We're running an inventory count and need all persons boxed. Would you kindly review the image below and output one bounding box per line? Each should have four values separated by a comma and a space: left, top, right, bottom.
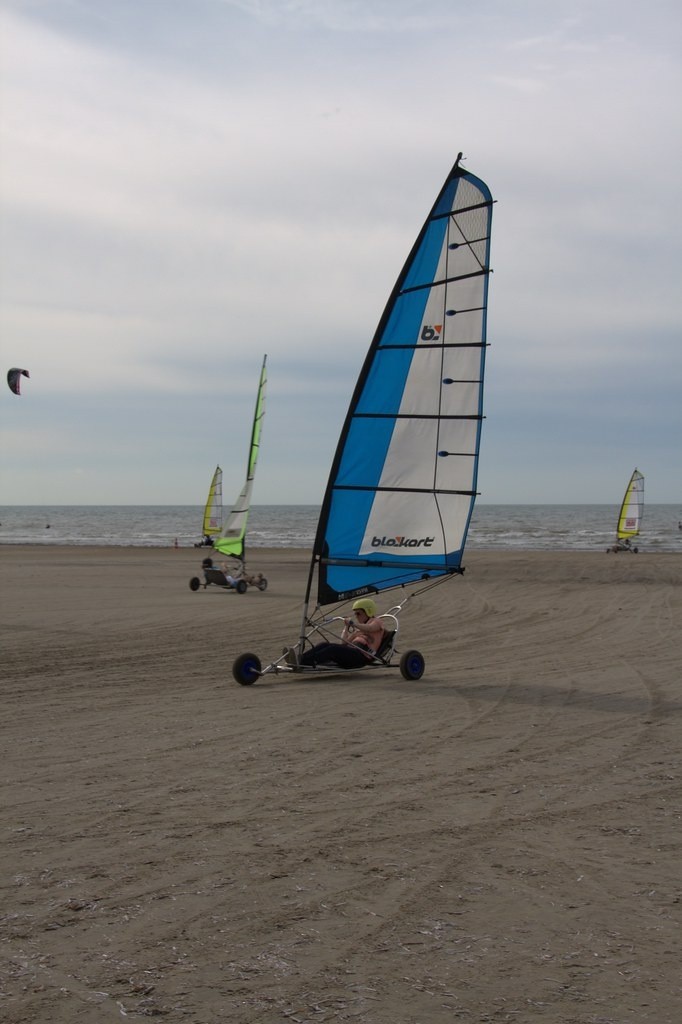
201, 556, 264, 588
197, 535, 211, 547
611, 539, 631, 552
283, 598, 387, 672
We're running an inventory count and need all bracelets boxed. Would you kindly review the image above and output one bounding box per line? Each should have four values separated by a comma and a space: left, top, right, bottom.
348, 619, 355, 627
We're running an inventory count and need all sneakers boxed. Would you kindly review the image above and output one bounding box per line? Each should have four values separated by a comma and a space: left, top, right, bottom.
282, 646, 299, 669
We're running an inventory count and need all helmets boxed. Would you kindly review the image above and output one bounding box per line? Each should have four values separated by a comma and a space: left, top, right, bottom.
353, 599, 376, 618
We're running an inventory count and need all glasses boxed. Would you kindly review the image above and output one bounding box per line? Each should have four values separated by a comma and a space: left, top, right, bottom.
354, 611, 365, 616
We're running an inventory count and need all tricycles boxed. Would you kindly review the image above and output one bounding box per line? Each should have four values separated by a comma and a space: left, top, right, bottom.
605, 466, 644, 552
193, 464, 222, 549
189, 354, 269, 591
218, 152, 500, 684
